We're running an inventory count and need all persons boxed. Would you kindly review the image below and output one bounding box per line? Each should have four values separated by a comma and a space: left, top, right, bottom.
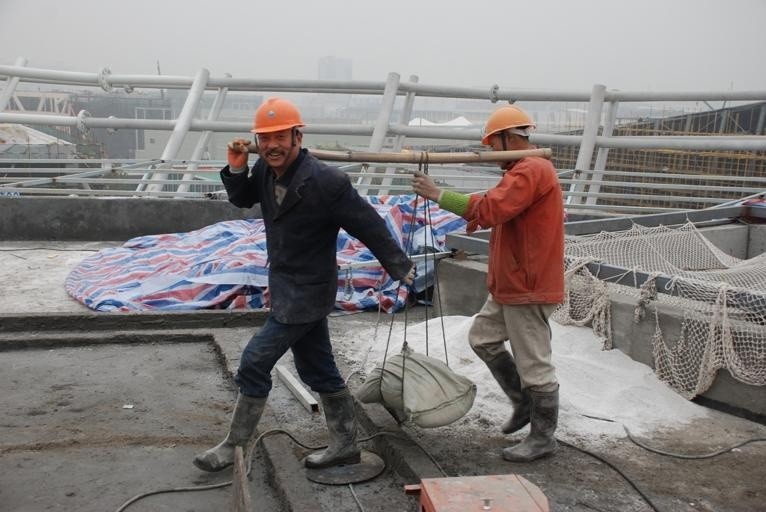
193, 97, 416, 471
412, 106, 565, 462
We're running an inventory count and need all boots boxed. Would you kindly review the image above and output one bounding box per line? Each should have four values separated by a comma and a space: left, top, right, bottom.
305, 385, 360, 469
193, 388, 268, 472
485, 351, 530, 433
502, 384, 560, 462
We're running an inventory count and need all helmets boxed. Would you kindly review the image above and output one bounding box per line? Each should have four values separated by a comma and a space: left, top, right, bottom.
482, 106, 535, 144
250, 97, 305, 133
